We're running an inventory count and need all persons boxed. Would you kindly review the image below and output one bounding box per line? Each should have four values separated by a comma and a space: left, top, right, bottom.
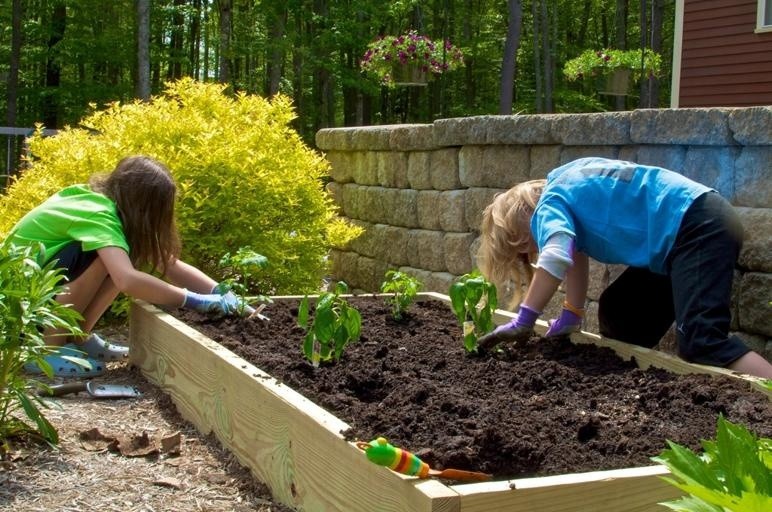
466, 157, 771, 385
1, 154, 272, 379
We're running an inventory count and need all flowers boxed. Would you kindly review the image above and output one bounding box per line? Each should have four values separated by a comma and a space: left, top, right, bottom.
359, 29, 467, 90
562, 47, 664, 82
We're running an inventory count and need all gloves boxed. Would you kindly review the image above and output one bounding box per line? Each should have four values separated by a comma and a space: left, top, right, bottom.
479, 304, 543, 340
545, 301, 587, 336
181, 284, 268, 320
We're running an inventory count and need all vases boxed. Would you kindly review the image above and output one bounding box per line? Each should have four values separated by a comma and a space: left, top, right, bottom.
605, 70, 629, 94
392, 64, 426, 83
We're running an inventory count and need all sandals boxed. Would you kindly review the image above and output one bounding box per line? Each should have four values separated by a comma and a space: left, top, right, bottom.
26, 333, 129, 377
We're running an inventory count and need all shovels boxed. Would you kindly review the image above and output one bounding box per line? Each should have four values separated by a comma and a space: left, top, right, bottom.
358, 438, 494, 482
37, 381, 141, 399
